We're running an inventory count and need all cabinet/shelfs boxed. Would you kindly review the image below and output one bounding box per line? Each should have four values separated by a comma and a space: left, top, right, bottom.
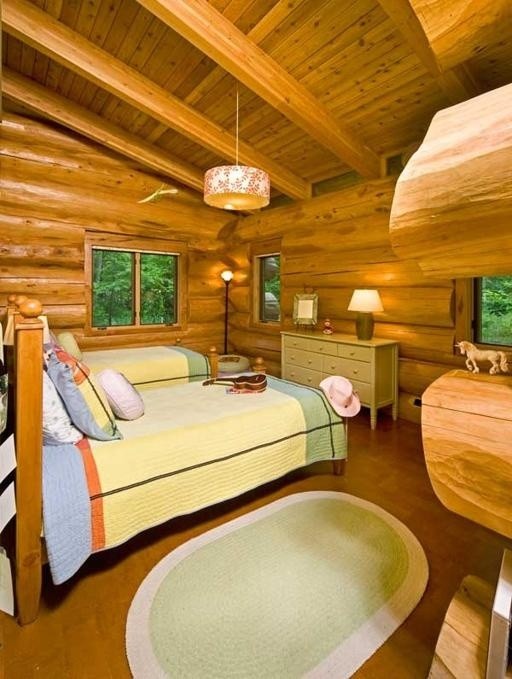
278, 331, 400, 431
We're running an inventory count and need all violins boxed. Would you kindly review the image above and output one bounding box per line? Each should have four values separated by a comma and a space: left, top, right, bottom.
203, 375, 267, 389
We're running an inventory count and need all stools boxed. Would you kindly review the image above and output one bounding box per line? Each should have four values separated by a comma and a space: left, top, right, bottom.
215, 354, 251, 378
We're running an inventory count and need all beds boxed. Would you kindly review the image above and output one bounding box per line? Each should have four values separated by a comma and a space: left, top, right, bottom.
12, 295, 346, 627
6, 294, 219, 396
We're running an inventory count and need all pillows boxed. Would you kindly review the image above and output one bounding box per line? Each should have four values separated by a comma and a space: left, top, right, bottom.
57, 332, 83, 362
50, 329, 59, 344
41, 343, 125, 441
97, 368, 145, 421
42, 370, 84, 446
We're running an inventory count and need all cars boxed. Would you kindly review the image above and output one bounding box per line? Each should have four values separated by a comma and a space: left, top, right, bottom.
265, 292, 279, 321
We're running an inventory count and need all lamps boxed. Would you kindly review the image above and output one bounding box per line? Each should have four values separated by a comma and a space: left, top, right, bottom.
346, 289, 384, 342
220, 270, 234, 353
203, 85, 271, 211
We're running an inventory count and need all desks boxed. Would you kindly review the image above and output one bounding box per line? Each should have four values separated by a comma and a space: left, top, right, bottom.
420, 371, 511, 538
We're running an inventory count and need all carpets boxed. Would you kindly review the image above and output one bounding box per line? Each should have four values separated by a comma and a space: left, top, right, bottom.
125, 489, 430, 679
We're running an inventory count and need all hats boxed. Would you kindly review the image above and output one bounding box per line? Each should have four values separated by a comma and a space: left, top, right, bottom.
318, 375, 361, 418
